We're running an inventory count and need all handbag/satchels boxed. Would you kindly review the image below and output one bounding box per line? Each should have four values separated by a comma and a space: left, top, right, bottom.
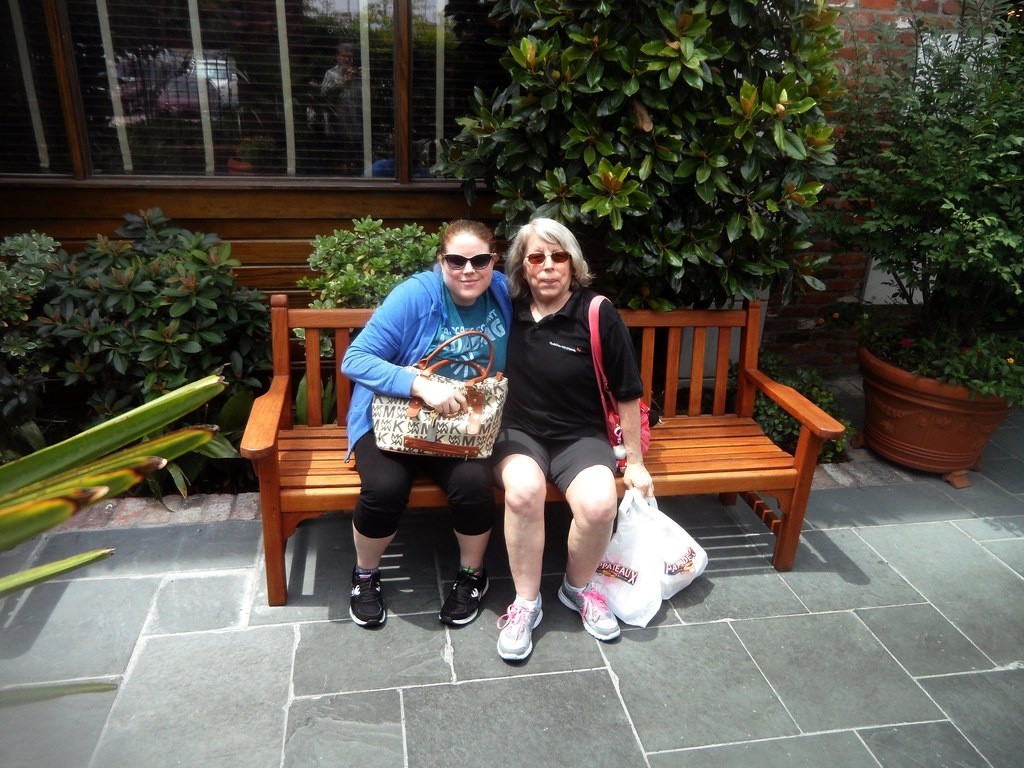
591, 488, 710, 627
372, 331, 508, 459
588, 295, 650, 473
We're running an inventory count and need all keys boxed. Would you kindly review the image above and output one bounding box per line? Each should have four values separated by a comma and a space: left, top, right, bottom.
429, 411, 439, 427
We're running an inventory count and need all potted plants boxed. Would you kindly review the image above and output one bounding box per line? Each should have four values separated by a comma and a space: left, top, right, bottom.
830, 0, 1024, 474
226, 137, 276, 174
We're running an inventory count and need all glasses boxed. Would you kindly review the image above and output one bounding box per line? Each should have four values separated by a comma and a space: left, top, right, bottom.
441, 253, 493, 270
524, 252, 571, 264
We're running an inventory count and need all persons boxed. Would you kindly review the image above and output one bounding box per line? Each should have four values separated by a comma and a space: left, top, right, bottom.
489, 216, 655, 660
319, 41, 366, 143
340, 218, 515, 627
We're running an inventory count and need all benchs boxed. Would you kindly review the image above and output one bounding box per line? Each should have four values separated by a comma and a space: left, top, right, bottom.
241, 297, 847, 608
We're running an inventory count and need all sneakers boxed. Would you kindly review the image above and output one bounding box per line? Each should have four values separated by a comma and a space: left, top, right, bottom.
558, 573, 621, 644
438, 566, 490, 630
349, 561, 386, 629
497, 591, 544, 665
